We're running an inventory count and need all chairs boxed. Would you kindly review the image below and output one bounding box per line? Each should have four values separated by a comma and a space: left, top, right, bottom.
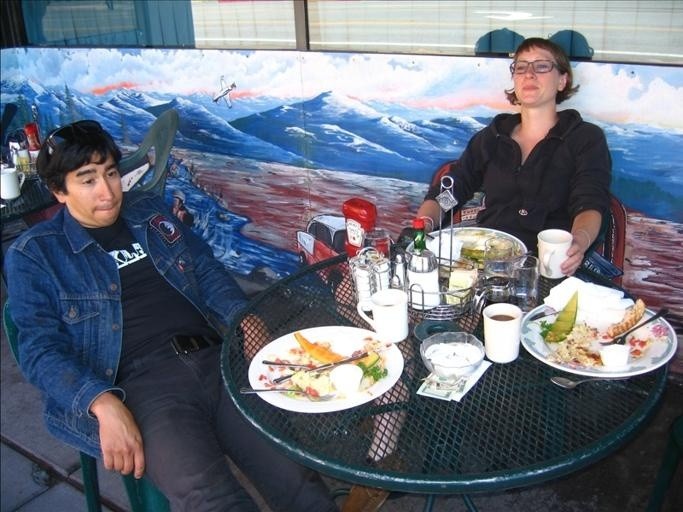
111, 107, 180, 201
429, 157, 627, 292
1, 291, 173, 511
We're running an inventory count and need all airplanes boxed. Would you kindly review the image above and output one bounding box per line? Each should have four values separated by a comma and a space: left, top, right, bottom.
210, 73, 236, 109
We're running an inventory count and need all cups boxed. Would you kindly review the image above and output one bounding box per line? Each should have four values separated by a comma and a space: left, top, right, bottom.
481, 237, 540, 308
330, 363, 364, 398
482, 303, 522, 365
538, 228, 572, 280
598, 344, 630, 367
356, 288, 408, 344
0, 167, 25, 201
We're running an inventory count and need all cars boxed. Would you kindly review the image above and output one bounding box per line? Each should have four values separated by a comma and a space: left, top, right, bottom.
294, 209, 392, 291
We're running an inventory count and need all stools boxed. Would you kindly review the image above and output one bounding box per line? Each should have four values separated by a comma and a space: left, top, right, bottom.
643, 414, 682, 512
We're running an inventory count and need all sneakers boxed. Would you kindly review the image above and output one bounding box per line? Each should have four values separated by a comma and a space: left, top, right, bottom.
357, 413, 376, 435
337, 448, 413, 512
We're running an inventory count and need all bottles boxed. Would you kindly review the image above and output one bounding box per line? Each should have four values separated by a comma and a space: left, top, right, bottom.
349, 218, 440, 311
7, 121, 44, 178
340, 197, 378, 260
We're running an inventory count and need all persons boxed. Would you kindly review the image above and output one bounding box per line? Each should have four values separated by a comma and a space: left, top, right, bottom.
3, 118, 337, 512
416, 37, 614, 277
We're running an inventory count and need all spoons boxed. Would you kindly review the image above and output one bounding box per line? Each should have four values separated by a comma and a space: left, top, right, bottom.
548, 374, 601, 389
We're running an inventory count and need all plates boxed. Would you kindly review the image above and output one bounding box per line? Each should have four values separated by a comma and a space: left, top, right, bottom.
246, 324, 406, 412
518, 303, 678, 379
403, 227, 529, 272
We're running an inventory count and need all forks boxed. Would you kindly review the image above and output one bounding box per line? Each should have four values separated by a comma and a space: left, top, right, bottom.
238, 387, 334, 402
600, 308, 668, 346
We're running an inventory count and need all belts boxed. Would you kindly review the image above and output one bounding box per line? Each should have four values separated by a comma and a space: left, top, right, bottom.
112, 326, 227, 385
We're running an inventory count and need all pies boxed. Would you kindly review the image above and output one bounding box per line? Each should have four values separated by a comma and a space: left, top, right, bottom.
606, 298, 646, 338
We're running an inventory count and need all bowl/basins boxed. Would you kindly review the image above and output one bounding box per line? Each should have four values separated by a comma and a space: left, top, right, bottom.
418, 331, 484, 378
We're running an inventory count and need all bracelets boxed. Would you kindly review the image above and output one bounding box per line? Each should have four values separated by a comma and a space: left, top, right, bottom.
419, 215, 434, 229
573, 229, 591, 248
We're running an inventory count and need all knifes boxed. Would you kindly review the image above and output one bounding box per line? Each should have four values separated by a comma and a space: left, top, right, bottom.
529, 306, 560, 321
272, 349, 372, 383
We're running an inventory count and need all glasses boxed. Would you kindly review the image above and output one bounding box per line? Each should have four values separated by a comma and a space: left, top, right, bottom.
41, 119, 107, 157
508, 57, 565, 77
